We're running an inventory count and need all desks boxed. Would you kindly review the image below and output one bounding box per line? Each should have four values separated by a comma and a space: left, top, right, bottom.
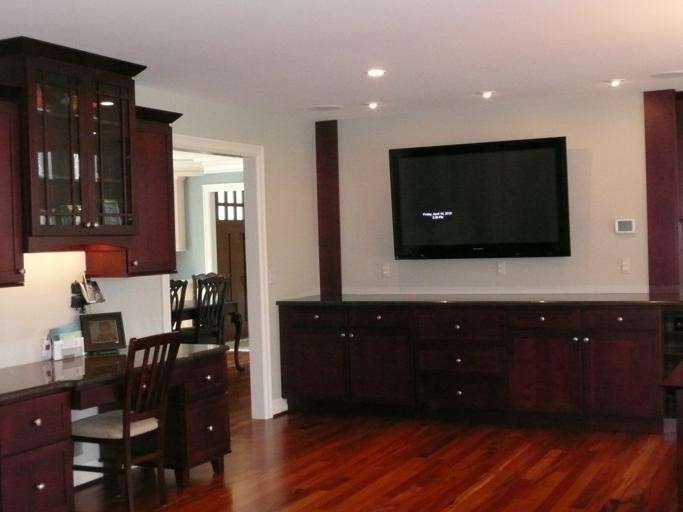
0, 340, 232, 510
169, 299, 245, 372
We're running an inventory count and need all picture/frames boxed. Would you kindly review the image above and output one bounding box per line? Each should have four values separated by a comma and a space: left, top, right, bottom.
77, 311, 126, 353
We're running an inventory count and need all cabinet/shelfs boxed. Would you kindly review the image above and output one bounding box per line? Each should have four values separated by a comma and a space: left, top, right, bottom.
275, 292, 683, 435
0, 36, 182, 290
0, 390, 74, 511
97, 354, 232, 487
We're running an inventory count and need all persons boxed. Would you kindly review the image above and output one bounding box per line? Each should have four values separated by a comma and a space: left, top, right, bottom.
94, 323, 113, 341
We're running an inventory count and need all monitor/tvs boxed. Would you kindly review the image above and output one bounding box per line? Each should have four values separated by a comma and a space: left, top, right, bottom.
390, 135, 572, 261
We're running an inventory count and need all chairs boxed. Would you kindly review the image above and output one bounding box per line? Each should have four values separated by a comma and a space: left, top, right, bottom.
169, 272, 231, 345
70, 329, 185, 511
